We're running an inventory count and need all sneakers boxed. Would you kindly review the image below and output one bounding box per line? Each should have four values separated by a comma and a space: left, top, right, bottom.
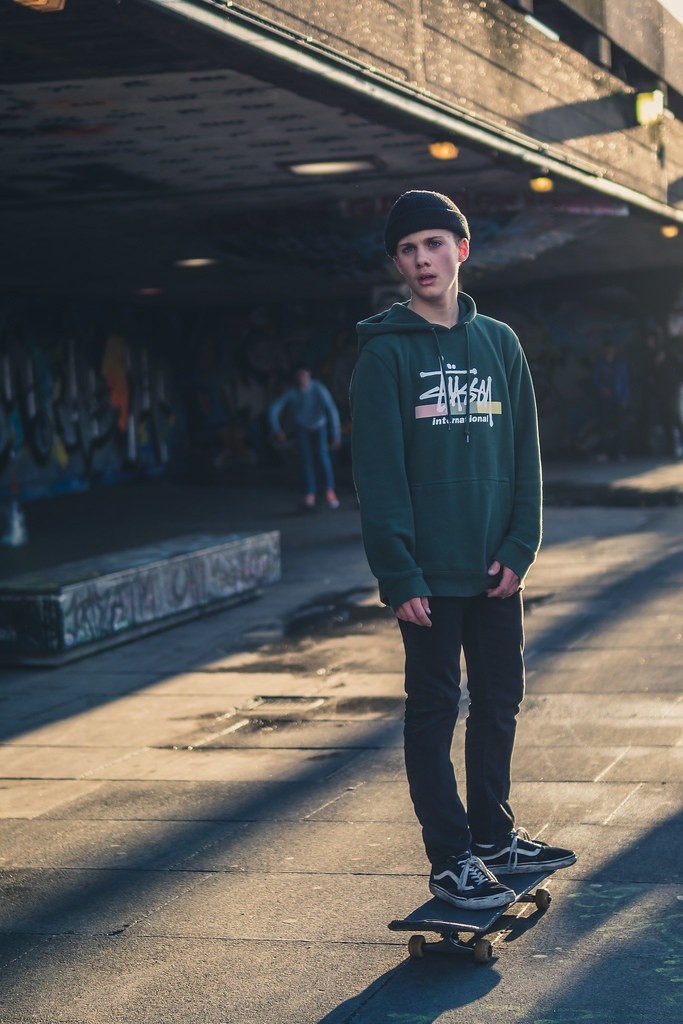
472, 828, 576, 875
428, 848, 515, 909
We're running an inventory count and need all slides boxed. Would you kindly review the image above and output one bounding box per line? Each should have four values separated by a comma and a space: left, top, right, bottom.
387, 840, 558, 963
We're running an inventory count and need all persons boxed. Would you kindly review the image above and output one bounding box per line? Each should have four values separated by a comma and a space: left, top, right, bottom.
268, 364, 342, 511
349, 190, 578, 910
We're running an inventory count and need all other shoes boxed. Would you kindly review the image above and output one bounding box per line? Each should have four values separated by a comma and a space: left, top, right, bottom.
327, 494, 338, 509
306, 494, 315, 507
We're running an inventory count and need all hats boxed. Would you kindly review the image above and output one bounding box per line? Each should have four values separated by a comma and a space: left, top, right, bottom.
385, 189, 470, 258
